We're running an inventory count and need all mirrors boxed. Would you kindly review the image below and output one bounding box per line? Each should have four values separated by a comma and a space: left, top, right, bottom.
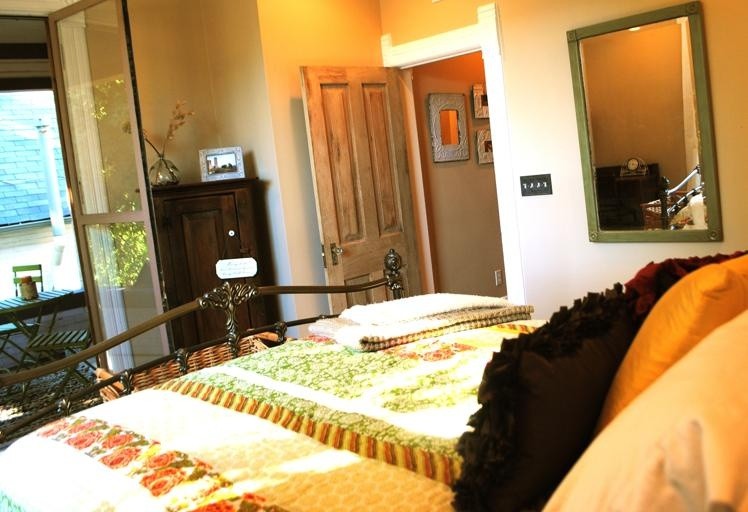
427, 90, 471, 164
565, 0, 721, 249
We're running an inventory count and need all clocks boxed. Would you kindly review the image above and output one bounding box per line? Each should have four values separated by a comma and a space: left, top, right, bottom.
619, 157, 649, 180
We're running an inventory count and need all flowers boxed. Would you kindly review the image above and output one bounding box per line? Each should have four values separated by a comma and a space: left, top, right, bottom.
120, 95, 199, 184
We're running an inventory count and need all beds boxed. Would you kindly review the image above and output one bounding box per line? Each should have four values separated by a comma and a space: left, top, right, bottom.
0, 249, 550, 511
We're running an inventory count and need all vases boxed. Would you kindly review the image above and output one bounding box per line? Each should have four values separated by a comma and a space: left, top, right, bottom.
150, 155, 180, 188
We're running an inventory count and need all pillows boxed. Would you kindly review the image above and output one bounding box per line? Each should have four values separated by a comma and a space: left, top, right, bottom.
540, 310, 748, 511
599, 255, 745, 427
446, 250, 733, 510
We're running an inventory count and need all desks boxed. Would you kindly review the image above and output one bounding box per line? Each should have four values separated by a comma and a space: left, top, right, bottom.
595, 175, 664, 229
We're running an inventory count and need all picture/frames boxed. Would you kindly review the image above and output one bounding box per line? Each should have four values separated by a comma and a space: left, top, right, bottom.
475, 126, 494, 164
197, 146, 247, 184
471, 82, 491, 120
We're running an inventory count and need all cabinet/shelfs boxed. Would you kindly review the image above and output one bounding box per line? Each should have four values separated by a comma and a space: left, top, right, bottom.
149, 178, 285, 350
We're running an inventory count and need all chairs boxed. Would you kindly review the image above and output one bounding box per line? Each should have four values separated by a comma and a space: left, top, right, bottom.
0, 259, 110, 450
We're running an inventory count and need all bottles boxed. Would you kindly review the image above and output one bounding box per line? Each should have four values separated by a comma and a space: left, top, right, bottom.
19, 276, 38, 300
